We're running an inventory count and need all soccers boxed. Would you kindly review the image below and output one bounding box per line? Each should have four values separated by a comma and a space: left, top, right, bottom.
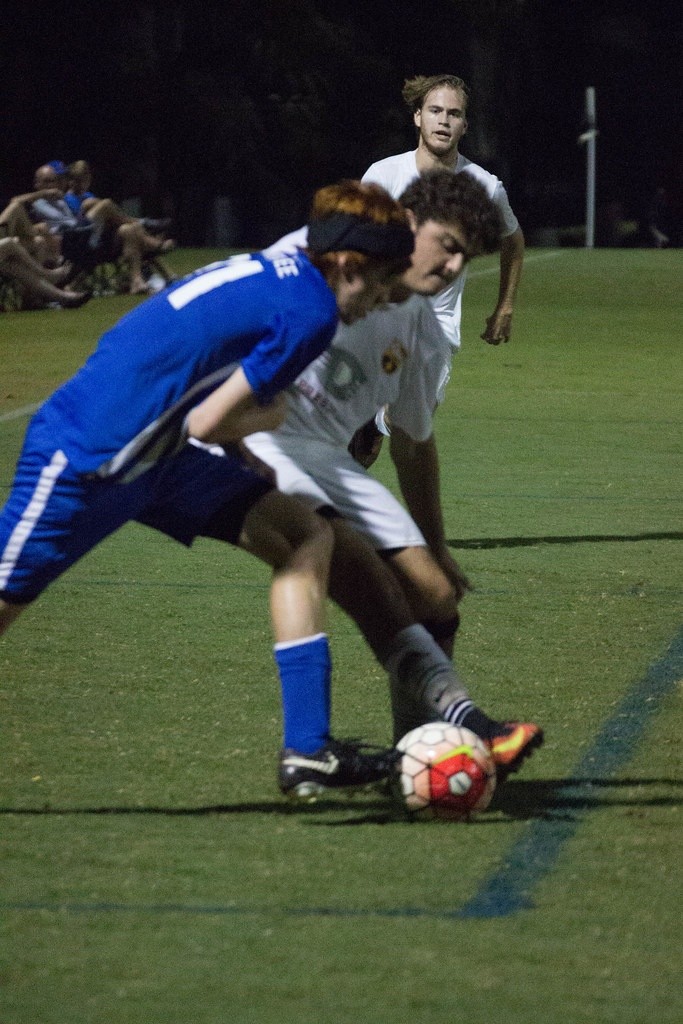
388, 720, 498, 820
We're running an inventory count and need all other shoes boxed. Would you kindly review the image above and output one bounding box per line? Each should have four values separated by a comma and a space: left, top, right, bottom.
150, 239, 176, 257
130, 277, 148, 293
57, 260, 85, 288
144, 217, 173, 235
62, 289, 92, 308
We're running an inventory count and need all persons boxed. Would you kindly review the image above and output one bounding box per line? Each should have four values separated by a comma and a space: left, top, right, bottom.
243, 167, 546, 789
1, 182, 416, 805
0, 159, 176, 314
345, 75, 526, 599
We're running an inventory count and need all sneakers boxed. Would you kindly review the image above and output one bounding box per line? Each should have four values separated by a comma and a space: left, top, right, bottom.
352, 418, 383, 469
485, 720, 544, 786
276, 738, 396, 807
